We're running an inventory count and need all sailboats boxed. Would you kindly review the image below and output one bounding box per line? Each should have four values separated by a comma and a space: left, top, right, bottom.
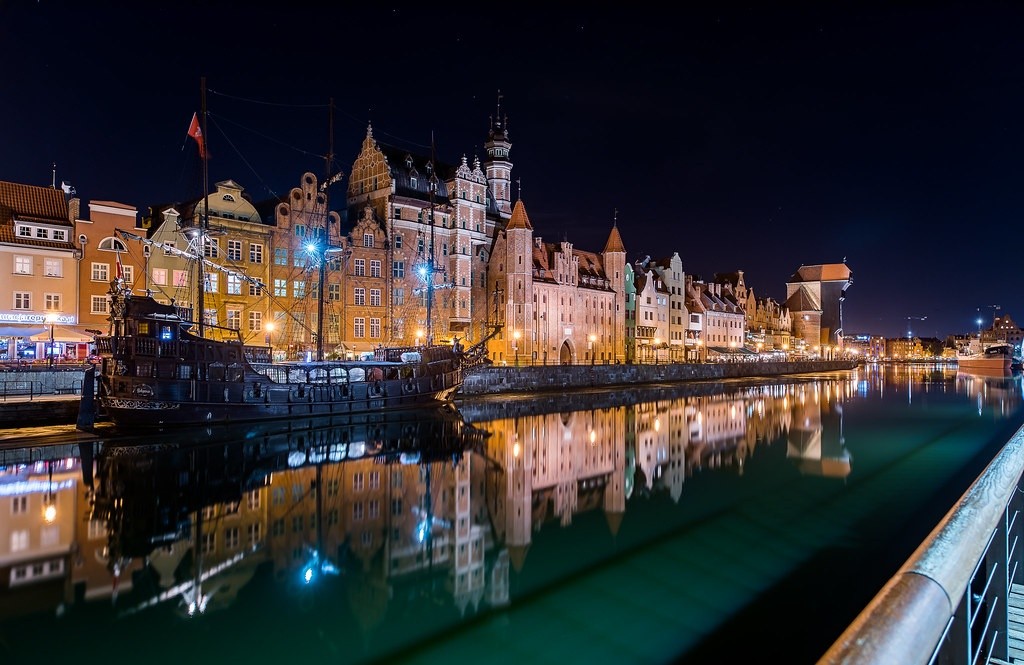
75, 77, 505, 446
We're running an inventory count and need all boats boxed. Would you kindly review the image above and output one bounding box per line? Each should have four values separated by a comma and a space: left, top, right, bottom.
92, 399, 503, 609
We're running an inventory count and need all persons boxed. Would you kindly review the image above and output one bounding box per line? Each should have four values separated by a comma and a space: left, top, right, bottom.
60, 346, 73, 361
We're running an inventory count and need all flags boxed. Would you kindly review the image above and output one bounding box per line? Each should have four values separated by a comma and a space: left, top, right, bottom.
115, 255, 122, 280
188, 116, 210, 159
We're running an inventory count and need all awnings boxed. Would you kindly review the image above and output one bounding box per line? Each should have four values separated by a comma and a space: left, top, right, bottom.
708, 347, 759, 356
0, 327, 45, 336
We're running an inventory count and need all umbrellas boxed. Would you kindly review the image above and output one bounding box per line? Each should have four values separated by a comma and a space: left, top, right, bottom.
30, 328, 95, 363
